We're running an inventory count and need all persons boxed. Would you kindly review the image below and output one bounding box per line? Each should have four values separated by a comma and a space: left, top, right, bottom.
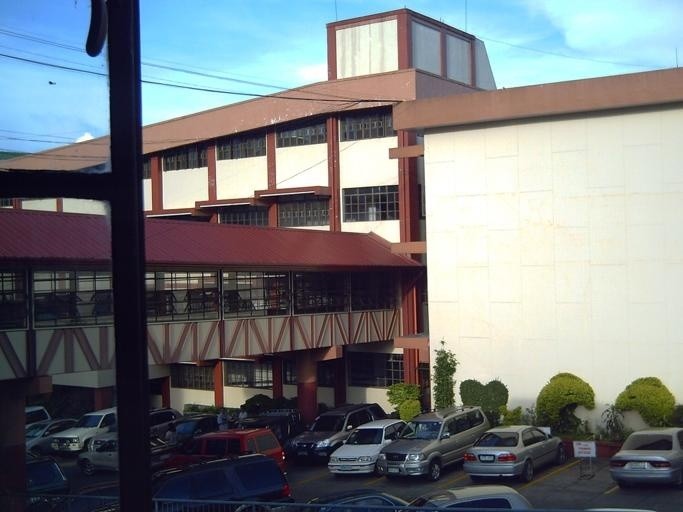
216, 407, 235, 431
238, 405, 248, 427
165, 423, 178, 441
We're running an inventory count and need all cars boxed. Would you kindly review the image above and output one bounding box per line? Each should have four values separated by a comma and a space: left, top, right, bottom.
16, 395, 305, 476
298, 491, 410, 512
609, 428, 682, 491
328, 420, 415, 474
462, 425, 567, 483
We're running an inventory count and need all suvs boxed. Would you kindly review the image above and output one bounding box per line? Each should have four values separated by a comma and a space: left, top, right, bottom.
145, 454, 294, 512
376, 405, 493, 479
397, 484, 533, 510
290, 404, 389, 460
163, 426, 287, 471
